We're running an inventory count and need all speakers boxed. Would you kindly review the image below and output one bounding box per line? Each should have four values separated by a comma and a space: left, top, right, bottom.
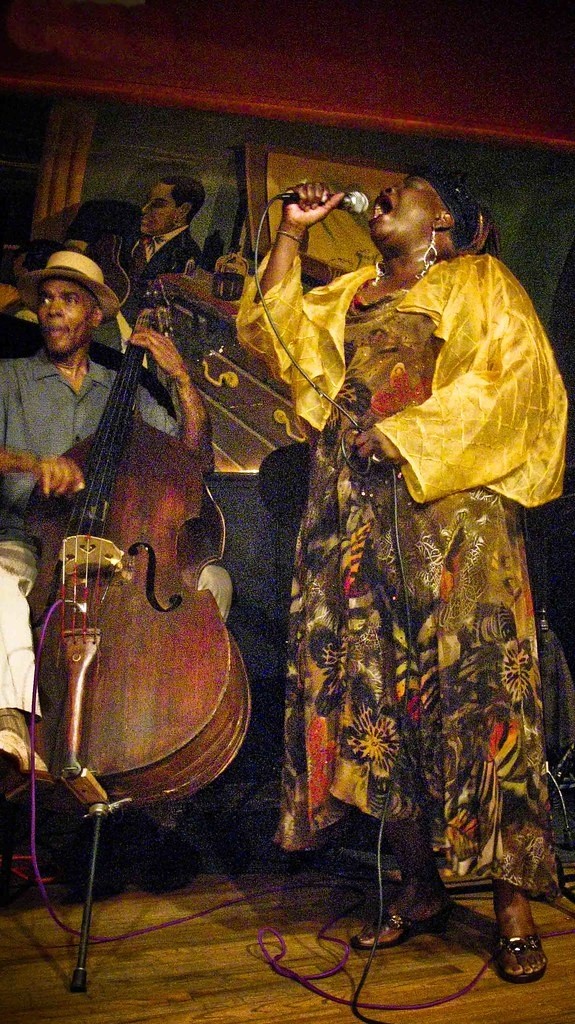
185, 470, 301, 812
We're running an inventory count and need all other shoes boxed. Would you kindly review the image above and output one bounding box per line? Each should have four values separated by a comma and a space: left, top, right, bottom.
0, 729, 53, 807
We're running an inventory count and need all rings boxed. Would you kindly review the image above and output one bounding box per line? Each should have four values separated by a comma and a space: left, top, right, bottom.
372, 453, 385, 463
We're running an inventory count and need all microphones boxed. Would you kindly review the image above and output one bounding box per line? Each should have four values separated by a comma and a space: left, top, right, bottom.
276, 191, 370, 215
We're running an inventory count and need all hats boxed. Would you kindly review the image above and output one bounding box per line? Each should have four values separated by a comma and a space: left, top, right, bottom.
17, 250, 119, 325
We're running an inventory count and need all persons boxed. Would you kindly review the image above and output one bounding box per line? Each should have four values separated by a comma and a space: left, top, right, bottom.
0, 252, 233, 786
234, 167, 570, 983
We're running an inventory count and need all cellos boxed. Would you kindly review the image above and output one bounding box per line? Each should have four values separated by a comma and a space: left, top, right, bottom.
28, 279, 253, 993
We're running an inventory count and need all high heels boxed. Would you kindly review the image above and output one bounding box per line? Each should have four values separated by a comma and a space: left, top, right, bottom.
350, 899, 458, 950
493, 930, 548, 985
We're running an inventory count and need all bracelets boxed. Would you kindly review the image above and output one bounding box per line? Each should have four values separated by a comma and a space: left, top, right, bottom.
277, 229, 302, 243
